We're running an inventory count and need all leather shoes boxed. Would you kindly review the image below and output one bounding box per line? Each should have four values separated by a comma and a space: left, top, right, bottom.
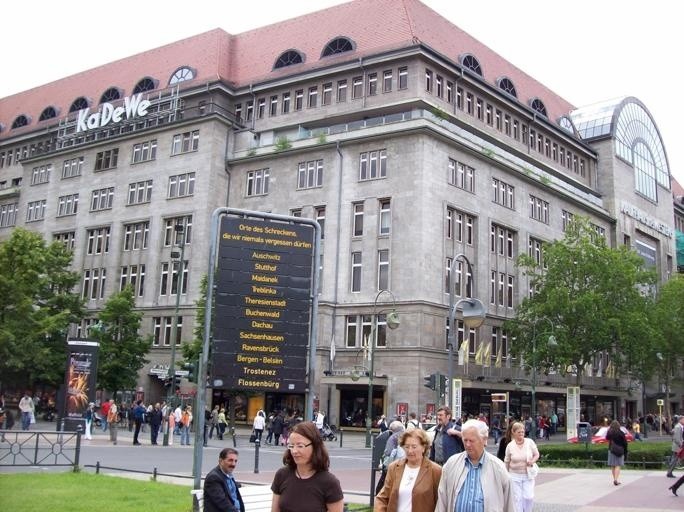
668, 475, 676, 478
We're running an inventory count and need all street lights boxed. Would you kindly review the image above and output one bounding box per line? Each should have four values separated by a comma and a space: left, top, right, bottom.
447, 250, 486, 418
368, 285, 400, 511
162, 216, 189, 405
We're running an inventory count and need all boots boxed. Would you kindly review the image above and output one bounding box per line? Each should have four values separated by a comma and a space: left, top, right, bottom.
669, 478, 684, 496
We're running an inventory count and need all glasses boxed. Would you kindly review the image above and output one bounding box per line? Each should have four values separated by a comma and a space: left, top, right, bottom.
288, 442, 313, 450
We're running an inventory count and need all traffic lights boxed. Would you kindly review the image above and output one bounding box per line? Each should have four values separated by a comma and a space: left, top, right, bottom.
164, 376, 172, 388
424, 374, 435, 390
444, 377, 448, 395
174, 374, 181, 392
398, 404, 406, 415
427, 404, 435, 413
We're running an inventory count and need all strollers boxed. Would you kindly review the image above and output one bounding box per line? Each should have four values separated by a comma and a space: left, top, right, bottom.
322, 423, 337, 442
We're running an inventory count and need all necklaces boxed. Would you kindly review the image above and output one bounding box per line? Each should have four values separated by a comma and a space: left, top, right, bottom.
296, 469, 302, 480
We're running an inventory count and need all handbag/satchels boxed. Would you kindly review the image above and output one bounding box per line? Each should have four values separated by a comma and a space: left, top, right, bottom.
610, 440, 624, 457
526, 462, 540, 480
250, 435, 256, 442
635, 432, 639, 439
383, 445, 406, 467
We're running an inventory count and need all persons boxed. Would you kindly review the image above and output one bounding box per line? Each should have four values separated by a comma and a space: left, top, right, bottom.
503, 422, 540, 512
666, 416, 684, 479
253, 406, 330, 446
632, 411, 666, 441
603, 419, 629, 486
372, 429, 442, 512
433, 418, 515, 512
32, 394, 40, 412
667, 475, 684, 497
84, 397, 230, 447
270, 420, 345, 512
18, 394, 38, 431
203, 448, 245, 512
374, 405, 559, 471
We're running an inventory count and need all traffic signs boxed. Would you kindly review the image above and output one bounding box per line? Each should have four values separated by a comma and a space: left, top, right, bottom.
206, 217, 314, 391
491, 393, 507, 402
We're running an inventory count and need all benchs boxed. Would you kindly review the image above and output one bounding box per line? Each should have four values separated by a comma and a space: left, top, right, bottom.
190, 484, 272, 512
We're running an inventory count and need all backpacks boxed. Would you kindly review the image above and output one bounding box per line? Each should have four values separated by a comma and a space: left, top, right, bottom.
410, 420, 419, 428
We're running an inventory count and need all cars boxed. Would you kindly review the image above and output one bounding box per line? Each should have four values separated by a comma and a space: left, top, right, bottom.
566, 425, 633, 446
